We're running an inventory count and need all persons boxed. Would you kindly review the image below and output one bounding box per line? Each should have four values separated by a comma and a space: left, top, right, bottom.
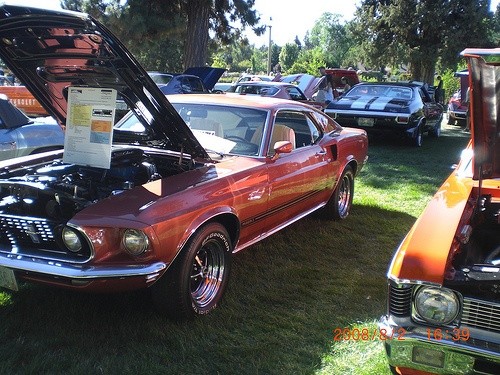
463, 87, 470, 132
436, 75, 443, 104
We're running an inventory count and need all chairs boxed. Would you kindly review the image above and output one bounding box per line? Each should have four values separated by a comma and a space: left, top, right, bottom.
189, 118, 223, 140
249, 123, 296, 156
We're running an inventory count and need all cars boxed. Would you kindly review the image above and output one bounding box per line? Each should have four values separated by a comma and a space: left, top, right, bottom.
0, 65, 473, 148
380, 45, 500, 375
1, 3, 369, 319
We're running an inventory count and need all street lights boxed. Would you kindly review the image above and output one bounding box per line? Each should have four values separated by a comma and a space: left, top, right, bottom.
263, 25, 273, 75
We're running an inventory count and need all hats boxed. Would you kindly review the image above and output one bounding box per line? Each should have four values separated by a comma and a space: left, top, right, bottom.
318, 67, 325, 69
